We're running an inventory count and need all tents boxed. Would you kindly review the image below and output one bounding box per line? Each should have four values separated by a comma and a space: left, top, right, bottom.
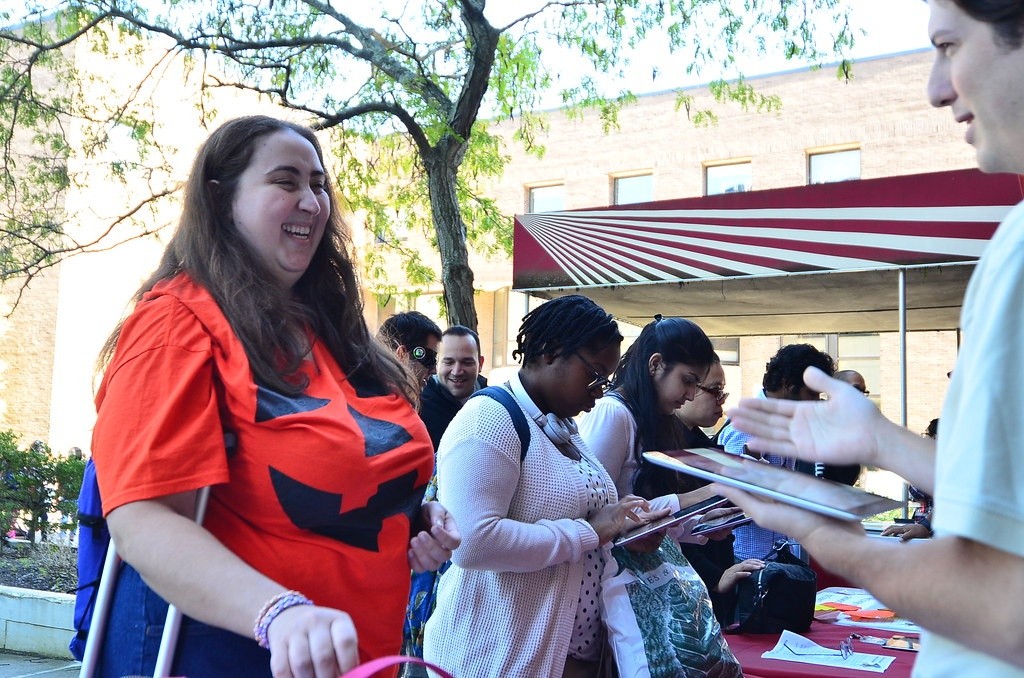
511, 167, 1024, 521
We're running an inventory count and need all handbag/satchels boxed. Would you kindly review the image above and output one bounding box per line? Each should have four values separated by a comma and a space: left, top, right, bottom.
603, 522, 744, 678
719, 539, 820, 634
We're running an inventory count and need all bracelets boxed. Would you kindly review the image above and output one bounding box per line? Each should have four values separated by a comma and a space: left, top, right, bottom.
919, 519, 935, 537
254, 590, 314, 649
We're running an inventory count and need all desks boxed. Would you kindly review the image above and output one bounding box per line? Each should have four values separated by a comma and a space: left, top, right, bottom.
726, 585, 923, 676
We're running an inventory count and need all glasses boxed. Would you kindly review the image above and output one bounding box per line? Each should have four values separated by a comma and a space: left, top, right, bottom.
698, 381, 725, 402
853, 384, 868, 393
920, 431, 928, 437
574, 350, 615, 395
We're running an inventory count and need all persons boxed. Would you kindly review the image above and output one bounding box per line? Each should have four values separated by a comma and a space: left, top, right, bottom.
91, 116, 461, 678
375, 313, 941, 630
421, 294, 723, 678
712, 0, 1024, 678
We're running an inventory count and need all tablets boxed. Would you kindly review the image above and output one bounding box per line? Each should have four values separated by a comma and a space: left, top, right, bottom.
691, 511, 754, 536
642, 446, 907, 522
615, 495, 729, 547
